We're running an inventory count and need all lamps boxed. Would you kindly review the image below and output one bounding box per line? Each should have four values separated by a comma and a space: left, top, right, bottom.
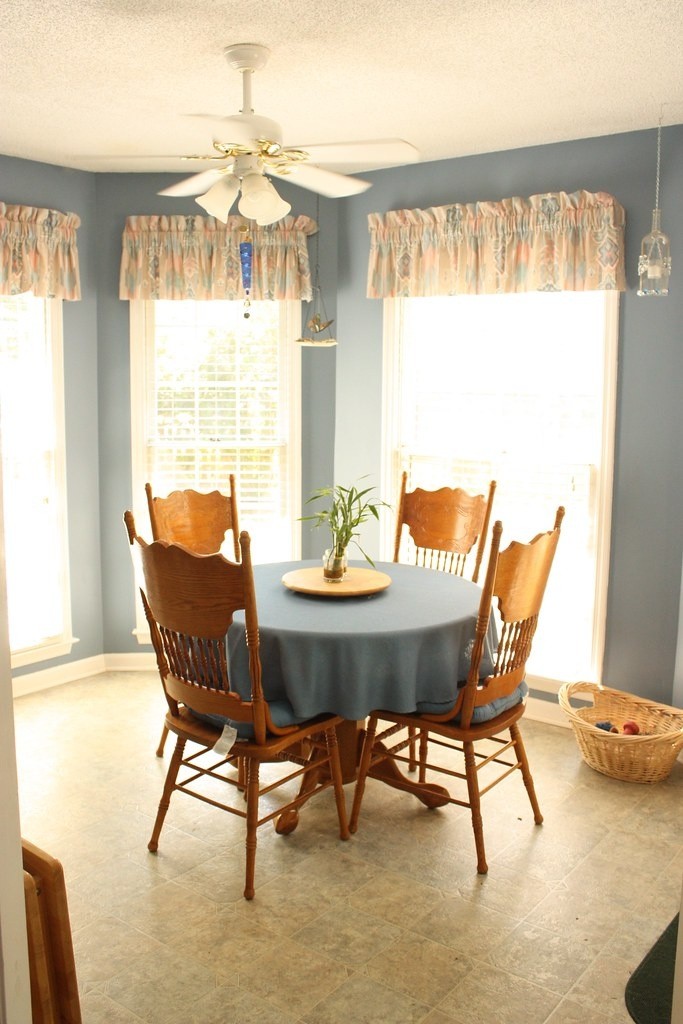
637, 116, 672, 297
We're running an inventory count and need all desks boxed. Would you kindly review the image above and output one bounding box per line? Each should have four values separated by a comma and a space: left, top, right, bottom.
173, 558, 498, 836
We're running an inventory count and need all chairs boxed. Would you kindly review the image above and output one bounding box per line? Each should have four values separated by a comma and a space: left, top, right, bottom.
123, 470, 565, 903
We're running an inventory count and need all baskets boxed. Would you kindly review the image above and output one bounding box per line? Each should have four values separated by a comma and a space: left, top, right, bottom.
558, 681, 683, 784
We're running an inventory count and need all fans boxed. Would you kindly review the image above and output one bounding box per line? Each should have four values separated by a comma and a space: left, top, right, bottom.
72, 40, 422, 227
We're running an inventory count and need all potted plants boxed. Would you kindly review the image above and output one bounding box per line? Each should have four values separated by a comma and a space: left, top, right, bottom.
296, 473, 392, 583
